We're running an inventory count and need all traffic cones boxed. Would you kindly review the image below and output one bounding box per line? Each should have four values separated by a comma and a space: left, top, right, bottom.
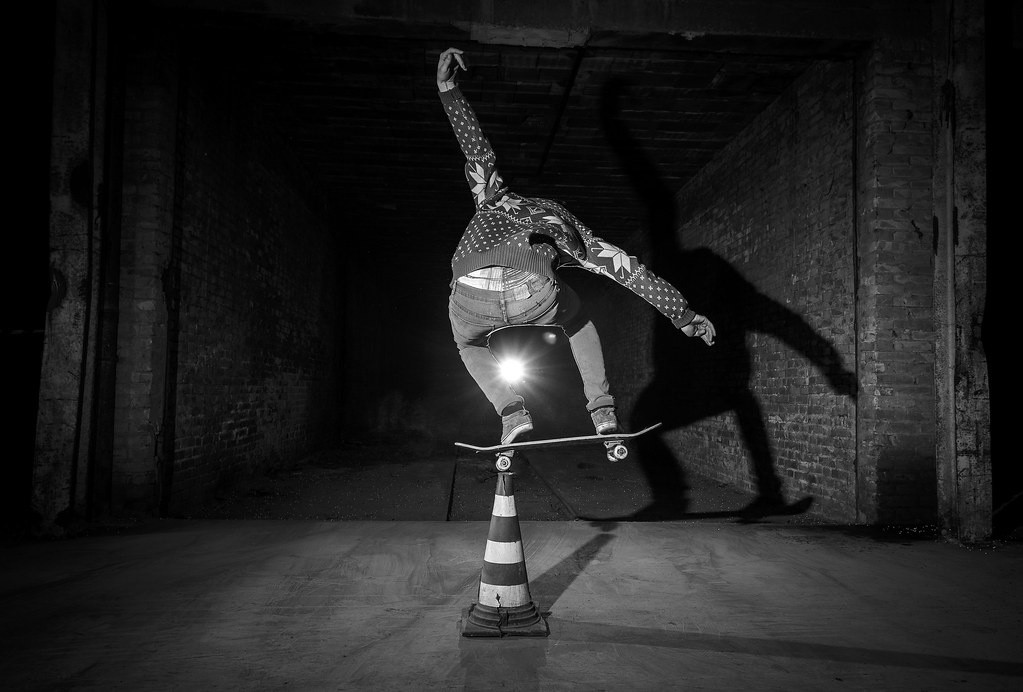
461, 470, 551, 638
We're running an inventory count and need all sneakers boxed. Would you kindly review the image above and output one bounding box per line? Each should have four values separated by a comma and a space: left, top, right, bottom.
590, 407, 617, 434
500, 410, 533, 445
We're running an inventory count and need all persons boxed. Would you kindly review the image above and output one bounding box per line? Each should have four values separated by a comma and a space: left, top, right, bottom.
435, 46, 716, 447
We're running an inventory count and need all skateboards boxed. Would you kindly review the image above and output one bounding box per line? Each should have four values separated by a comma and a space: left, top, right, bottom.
453, 420, 665, 472
